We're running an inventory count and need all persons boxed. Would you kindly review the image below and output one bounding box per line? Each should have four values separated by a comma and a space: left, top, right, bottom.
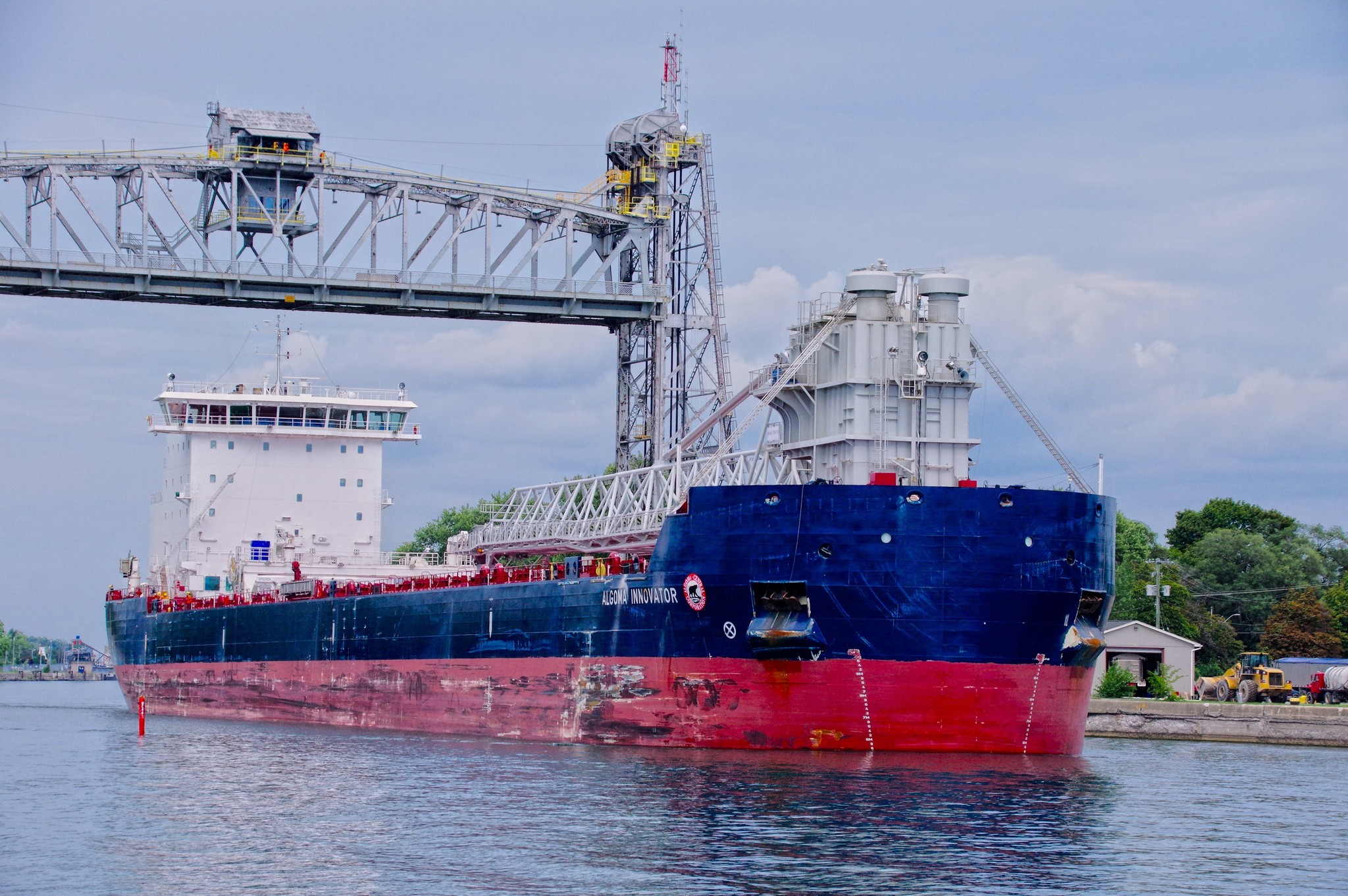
110, 585, 113, 593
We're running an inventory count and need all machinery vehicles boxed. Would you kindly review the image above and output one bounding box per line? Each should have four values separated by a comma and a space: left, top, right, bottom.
1195, 653, 1292, 703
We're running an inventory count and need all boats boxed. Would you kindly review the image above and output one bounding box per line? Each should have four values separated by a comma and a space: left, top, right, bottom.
101, 257, 1117, 754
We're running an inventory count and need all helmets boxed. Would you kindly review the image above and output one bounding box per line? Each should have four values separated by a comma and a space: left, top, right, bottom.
164, 593, 167, 596
186, 592, 190, 594
157, 592, 160, 595
109, 585, 112, 588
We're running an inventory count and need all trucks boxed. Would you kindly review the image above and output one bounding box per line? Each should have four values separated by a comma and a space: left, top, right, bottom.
1306, 671, 1348, 705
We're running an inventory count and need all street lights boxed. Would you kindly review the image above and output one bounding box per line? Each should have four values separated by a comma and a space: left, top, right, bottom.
1210, 606, 1240, 667
13, 637, 15, 673
32, 649, 36, 658
72, 648, 85, 673
